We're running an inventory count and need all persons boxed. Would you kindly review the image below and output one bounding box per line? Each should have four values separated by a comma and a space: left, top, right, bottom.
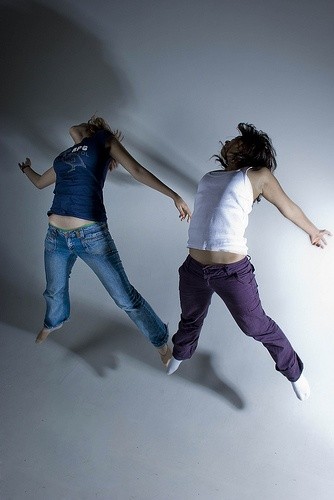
18, 110, 192, 364
166, 122, 333, 402
